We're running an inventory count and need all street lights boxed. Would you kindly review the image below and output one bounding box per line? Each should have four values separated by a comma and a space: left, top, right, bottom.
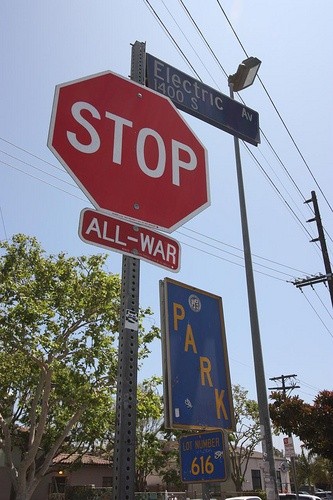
226, 57, 279, 500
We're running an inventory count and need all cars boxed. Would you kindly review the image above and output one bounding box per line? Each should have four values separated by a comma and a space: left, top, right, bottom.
225, 496, 262, 500
278, 493, 321, 500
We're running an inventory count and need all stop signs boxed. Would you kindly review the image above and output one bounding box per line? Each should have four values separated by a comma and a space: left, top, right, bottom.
47, 70, 212, 233
